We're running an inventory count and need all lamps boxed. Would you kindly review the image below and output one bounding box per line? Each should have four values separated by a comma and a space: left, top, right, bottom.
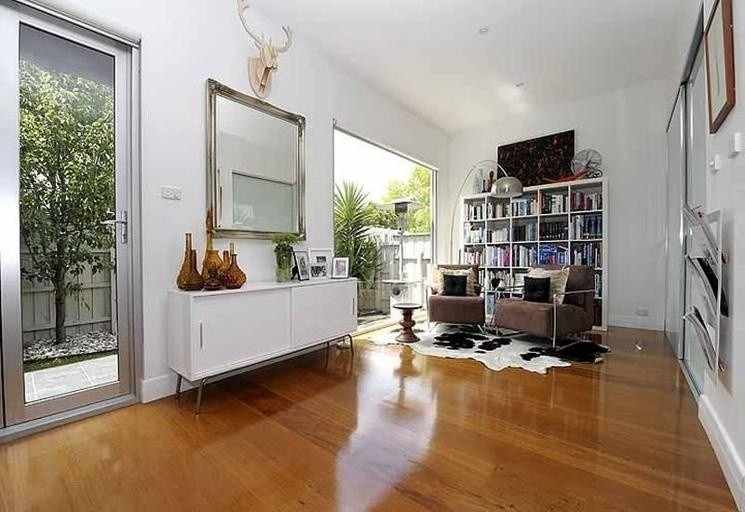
450, 160, 524, 265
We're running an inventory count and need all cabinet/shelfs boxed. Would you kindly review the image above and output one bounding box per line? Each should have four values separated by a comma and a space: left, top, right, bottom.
462, 176, 609, 331
167, 277, 358, 415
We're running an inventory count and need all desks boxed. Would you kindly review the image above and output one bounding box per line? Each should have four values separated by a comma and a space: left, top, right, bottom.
382, 280, 421, 320
393, 303, 423, 342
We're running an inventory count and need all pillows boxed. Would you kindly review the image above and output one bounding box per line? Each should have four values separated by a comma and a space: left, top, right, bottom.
439, 274, 468, 297
522, 276, 550, 303
527, 267, 570, 304
436, 267, 477, 297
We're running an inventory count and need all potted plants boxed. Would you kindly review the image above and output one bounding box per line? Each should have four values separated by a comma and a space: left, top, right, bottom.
271, 233, 300, 282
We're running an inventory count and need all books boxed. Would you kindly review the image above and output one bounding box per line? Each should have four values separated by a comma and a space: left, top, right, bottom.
678, 202, 730, 375
463, 190, 603, 327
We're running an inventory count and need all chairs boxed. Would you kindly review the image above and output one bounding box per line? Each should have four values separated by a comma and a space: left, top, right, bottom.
495, 264, 596, 354
429, 264, 486, 334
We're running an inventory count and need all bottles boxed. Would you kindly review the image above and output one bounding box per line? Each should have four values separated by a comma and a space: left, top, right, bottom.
175, 229, 247, 292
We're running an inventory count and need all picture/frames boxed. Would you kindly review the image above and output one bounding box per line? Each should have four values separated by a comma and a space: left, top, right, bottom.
332, 257, 350, 279
704, 0, 735, 132
291, 251, 309, 280
308, 247, 334, 264
308, 263, 332, 281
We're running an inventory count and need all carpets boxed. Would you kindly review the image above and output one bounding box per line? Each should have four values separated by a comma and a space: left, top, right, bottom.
352, 321, 612, 375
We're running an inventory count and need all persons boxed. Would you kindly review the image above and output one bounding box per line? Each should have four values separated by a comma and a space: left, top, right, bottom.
300, 258, 307, 275
336, 262, 346, 275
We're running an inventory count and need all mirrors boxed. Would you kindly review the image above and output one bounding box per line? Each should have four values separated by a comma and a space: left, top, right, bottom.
206, 77, 307, 242
